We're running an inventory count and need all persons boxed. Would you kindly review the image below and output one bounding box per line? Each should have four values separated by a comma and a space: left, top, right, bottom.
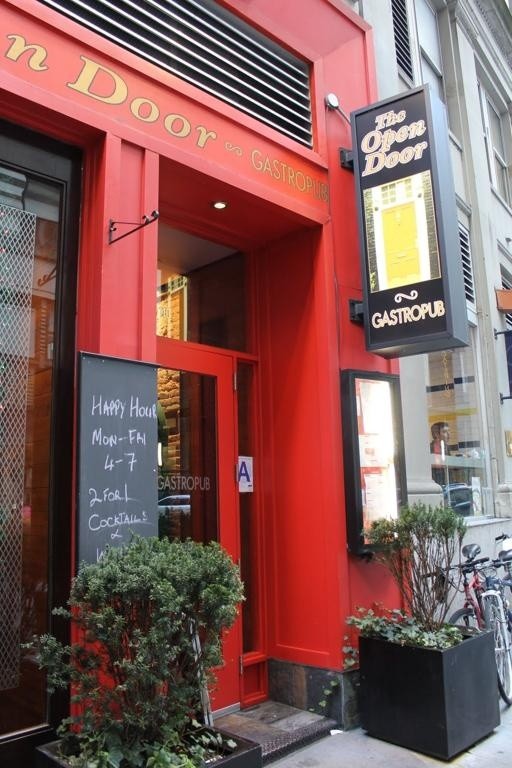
430, 421, 478, 487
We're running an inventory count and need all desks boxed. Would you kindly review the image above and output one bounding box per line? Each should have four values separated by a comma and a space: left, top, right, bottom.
430, 452, 485, 516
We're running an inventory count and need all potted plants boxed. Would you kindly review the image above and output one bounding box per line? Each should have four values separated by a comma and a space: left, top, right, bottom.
355, 502, 499, 763
24, 547, 261, 768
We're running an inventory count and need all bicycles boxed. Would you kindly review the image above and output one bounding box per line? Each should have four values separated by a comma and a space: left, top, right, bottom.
423, 527, 511, 706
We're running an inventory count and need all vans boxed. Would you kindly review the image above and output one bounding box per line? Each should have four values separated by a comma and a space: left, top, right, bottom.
441, 481, 476, 525
